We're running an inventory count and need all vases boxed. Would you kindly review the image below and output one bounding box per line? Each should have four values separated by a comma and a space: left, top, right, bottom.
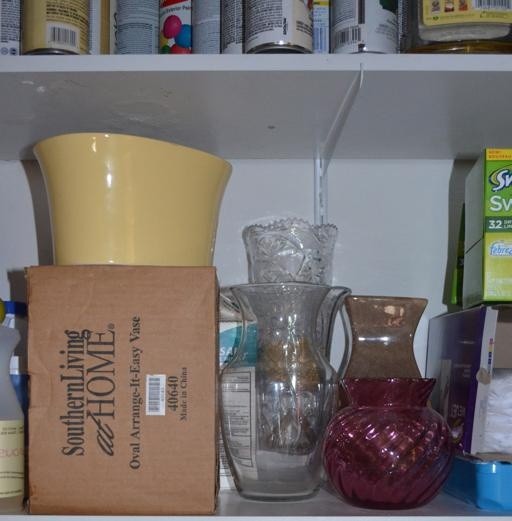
339, 294, 428, 377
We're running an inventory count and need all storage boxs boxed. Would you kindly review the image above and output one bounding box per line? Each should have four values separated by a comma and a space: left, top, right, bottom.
465, 149, 512, 310
443, 456, 512, 513
24, 265, 220, 516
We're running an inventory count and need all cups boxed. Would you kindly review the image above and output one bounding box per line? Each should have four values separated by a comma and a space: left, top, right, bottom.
28, 128, 232, 264
240, 218, 338, 453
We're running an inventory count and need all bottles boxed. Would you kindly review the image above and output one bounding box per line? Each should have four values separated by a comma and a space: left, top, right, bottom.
328, 292, 431, 411
0, 0, 510, 53
0, 299, 29, 513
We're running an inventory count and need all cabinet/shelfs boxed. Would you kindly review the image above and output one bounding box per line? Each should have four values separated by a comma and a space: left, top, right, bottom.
1, 52, 512, 520
